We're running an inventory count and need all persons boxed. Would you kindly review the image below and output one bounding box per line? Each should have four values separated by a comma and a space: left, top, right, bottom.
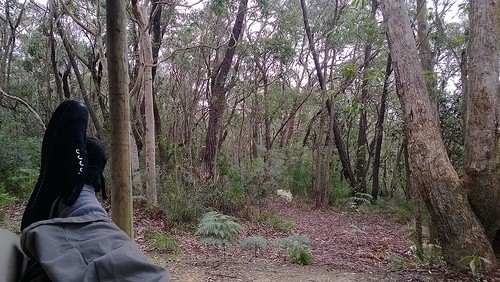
20, 99, 173, 282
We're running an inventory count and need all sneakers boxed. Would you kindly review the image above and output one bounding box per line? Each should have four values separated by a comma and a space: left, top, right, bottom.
20, 97, 109, 235
